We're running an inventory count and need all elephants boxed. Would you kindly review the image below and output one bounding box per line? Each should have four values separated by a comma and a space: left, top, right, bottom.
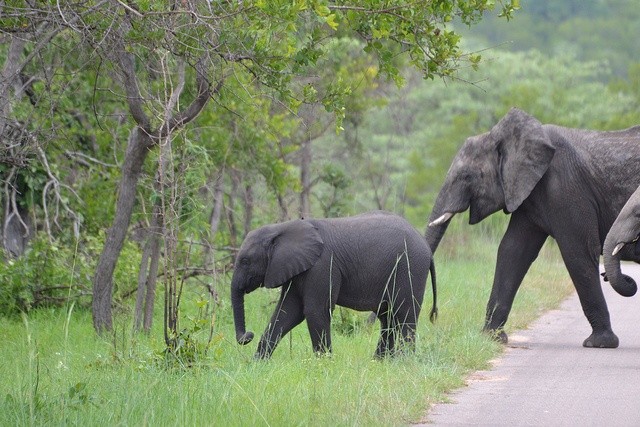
423, 105, 640, 348
602, 184, 640, 297
230, 210, 439, 361
